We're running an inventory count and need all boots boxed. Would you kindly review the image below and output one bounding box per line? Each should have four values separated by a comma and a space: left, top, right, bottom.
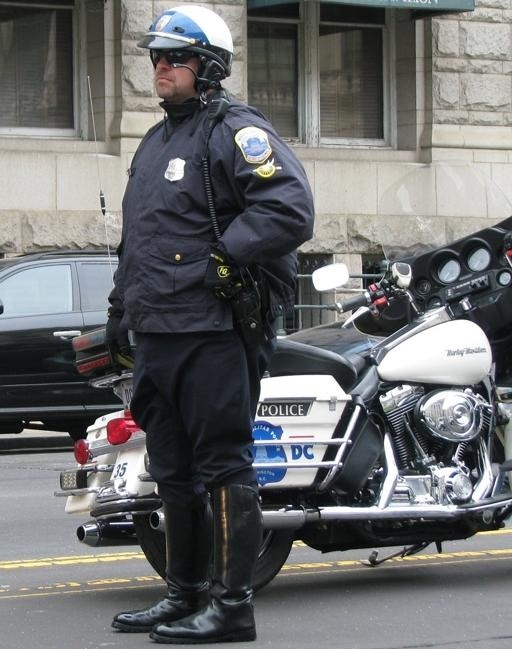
150, 483, 263, 641
111, 501, 213, 632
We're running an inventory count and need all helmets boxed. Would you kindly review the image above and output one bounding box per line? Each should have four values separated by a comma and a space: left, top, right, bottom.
137, 5, 233, 77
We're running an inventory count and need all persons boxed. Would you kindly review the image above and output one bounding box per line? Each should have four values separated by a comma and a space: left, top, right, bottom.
102, 6, 315, 645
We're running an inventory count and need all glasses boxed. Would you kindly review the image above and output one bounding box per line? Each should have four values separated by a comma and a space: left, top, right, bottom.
150, 49, 199, 63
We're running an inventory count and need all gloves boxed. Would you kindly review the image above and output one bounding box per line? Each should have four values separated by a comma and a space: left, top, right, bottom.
106, 306, 134, 376
203, 248, 243, 299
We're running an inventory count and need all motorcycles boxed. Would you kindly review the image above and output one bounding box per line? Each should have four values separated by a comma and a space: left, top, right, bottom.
52, 215, 512, 603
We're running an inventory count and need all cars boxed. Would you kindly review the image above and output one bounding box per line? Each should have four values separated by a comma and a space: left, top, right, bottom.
0, 247, 139, 446
